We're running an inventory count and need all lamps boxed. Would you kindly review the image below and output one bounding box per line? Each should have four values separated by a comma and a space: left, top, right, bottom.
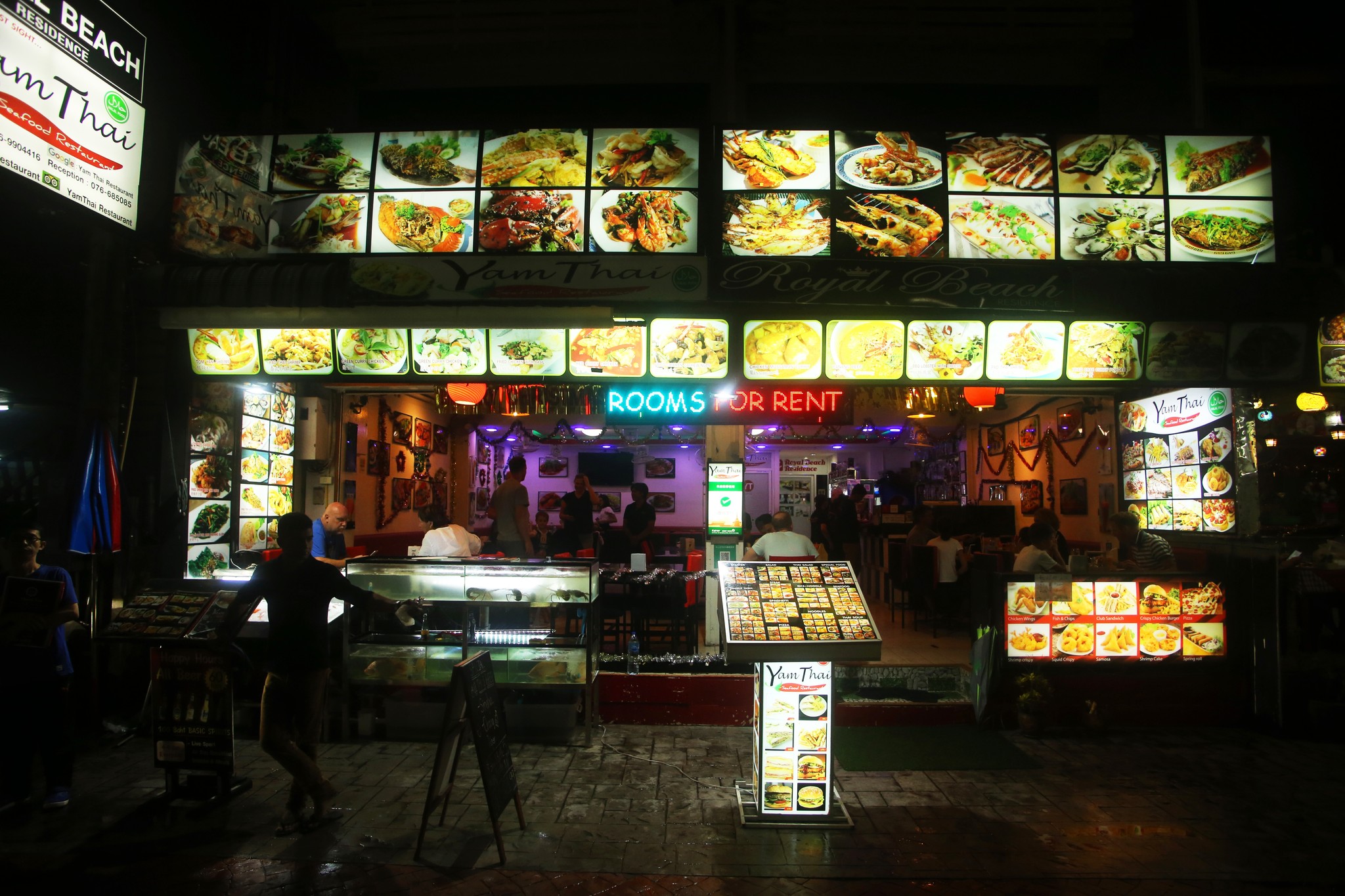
447, 384, 486, 405
963, 387, 1002, 407
906, 399, 936, 420
994, 387, 1008, 411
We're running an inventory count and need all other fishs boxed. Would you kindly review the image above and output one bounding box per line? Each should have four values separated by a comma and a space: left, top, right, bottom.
275, 138, 476, 250
1176, 133, 1274, 256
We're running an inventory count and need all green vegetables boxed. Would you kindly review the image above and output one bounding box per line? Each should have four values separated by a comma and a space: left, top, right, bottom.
1111, 323, 1144, 344
192, 504, 230, 540
188, 546, 228, 579
970, 201, 1034, 245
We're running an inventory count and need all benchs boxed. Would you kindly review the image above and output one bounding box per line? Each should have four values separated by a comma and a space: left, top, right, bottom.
352, 531, 428, 559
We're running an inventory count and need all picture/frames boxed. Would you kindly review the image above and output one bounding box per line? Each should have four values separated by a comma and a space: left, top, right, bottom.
1020, 483, 1042, 514
369, 410, 449, 510
1017, 413, 1041, 451
538, 457, 675, 513
988, 484, 1008, 502
985, 426, 1005, 457
475, 439, 507, 514
1059, 478, 1088, 514
1056, 401, 1084, 443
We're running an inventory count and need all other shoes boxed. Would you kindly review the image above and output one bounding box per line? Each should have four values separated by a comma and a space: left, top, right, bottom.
275, 805, 308, 837
310, 779, 337, 828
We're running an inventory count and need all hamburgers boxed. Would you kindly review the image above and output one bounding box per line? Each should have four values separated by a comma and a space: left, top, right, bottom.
798, 786, 824, 808
1140, 584, 1167, 613
798, 755, 824, 778
765, 785, 792, 808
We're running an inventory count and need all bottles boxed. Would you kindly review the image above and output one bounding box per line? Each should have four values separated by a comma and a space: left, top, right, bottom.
468, 611, 477, 642
421, 614, 430, 640
627, 631, 640, 674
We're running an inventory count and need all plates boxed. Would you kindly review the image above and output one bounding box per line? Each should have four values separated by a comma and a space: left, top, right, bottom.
1117, 399, 1237, 536
176, 132, 1277, 270
183, 384, 292, 576
798, 694, 829, 717
1008, 583, 1223, 657
110, 592, 229, 636
1110, 569, 1126, 572
187, 309, 1142, 380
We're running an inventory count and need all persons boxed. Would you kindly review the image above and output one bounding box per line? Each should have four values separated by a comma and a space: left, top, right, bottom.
838, 483, 874, 578
742, 514, 820, 561
821, 499, 845, 561
595, 493, 618, 564
1096, 512, 1177, 570
224, 513, 428, 828
486, 456, 534, 559
530, 511, 553, 559
559, 473, 599, 557
419, 503, 482, 630
743, 511, 752, 540
831, 489, 843, 501
927, 515, 968, 617
905, 505, 973, 578
593, 521, 604, 545
623, 483, 657, 565
312, 501, 370, 568
1013, 521, 1067, 574
1033, 508, 1070, 565
810, 494, 828, 543
746, 514, 773, 547
0, 518, 80, 810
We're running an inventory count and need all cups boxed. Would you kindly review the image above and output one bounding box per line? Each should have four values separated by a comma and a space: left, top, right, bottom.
1068, 549, 1080, 555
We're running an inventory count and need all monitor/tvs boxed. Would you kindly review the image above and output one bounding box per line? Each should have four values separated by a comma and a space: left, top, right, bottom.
960, 496, 967, 506
577, 452, 633, 486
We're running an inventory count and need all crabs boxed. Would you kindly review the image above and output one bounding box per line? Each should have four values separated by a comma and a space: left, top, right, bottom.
481, 127, 588, 253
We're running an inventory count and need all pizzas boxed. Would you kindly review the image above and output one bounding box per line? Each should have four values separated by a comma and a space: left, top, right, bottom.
1182, 582, 1223, 614
1123, 446, 1144, 470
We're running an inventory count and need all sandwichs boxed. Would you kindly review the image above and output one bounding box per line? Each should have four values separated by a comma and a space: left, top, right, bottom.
767, 731, 792, 747
765, 756, 793, 780
1098, 583, 1137, 613
767, 700, 794, 717
801, 696, 815, 711
798, 728, 826, 748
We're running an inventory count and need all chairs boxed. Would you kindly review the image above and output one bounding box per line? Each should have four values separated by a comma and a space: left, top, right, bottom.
885, 537, 1011, 645
553, 533, 705, 650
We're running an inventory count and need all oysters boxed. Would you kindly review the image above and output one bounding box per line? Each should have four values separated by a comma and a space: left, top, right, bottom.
1058, 136, 1167, 265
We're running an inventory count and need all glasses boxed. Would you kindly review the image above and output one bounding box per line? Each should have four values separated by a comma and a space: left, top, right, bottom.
13, 535, 43, 542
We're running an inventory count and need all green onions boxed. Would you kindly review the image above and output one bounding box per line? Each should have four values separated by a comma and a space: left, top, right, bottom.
1172, 212, 1261, 246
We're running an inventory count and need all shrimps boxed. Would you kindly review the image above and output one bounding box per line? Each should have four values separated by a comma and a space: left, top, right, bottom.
594, 126, 940, 256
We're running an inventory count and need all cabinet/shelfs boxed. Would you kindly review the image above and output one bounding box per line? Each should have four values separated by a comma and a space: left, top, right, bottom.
347, 554, 599, 741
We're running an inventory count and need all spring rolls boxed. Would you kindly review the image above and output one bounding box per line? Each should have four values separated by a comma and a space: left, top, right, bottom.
1148, 504, 1171, 525
1188, 632, 1213, 646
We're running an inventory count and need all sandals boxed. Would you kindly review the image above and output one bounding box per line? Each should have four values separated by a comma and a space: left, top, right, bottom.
42, 785, 73, 809
0, 789, 32, 811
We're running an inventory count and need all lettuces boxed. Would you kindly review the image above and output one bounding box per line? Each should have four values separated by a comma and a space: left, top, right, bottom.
440, 214, 465, 233
396, 204, 415, 220
